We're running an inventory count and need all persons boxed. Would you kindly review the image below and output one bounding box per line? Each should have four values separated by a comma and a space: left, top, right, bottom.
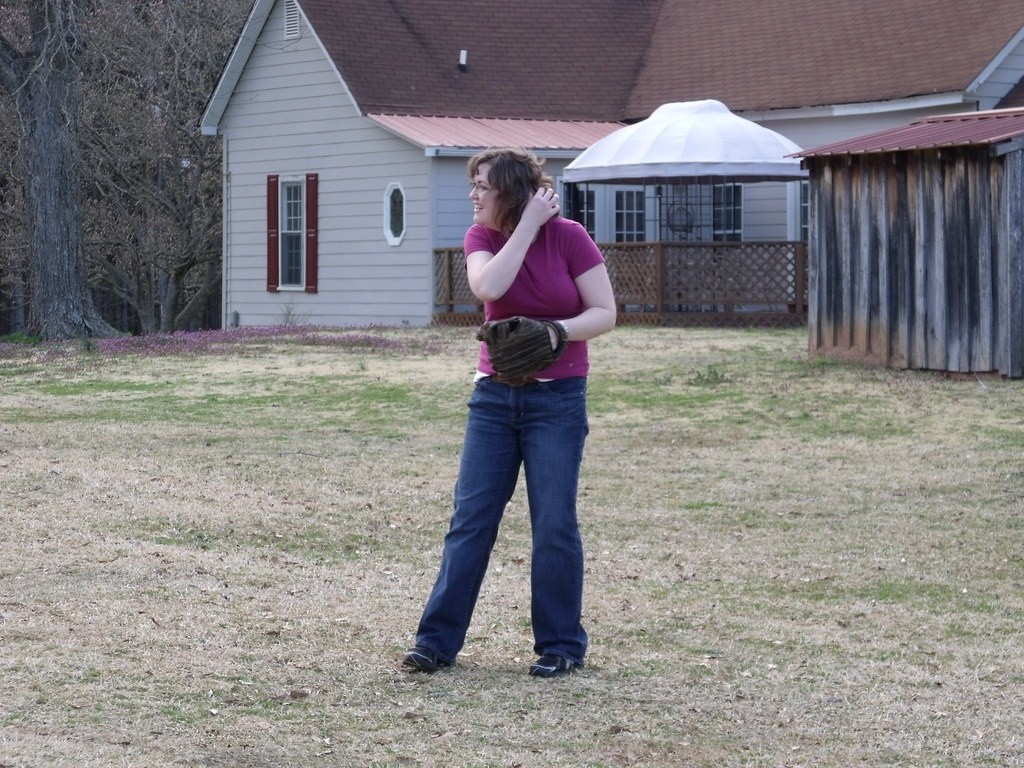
403, 146, 617, 676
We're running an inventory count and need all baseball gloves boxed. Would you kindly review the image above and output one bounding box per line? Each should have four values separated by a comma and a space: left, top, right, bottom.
476, 314, 568, 389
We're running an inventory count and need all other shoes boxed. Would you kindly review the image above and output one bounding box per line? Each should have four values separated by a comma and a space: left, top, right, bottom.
528, 653, 577, 678
402, 646, 447, 672
